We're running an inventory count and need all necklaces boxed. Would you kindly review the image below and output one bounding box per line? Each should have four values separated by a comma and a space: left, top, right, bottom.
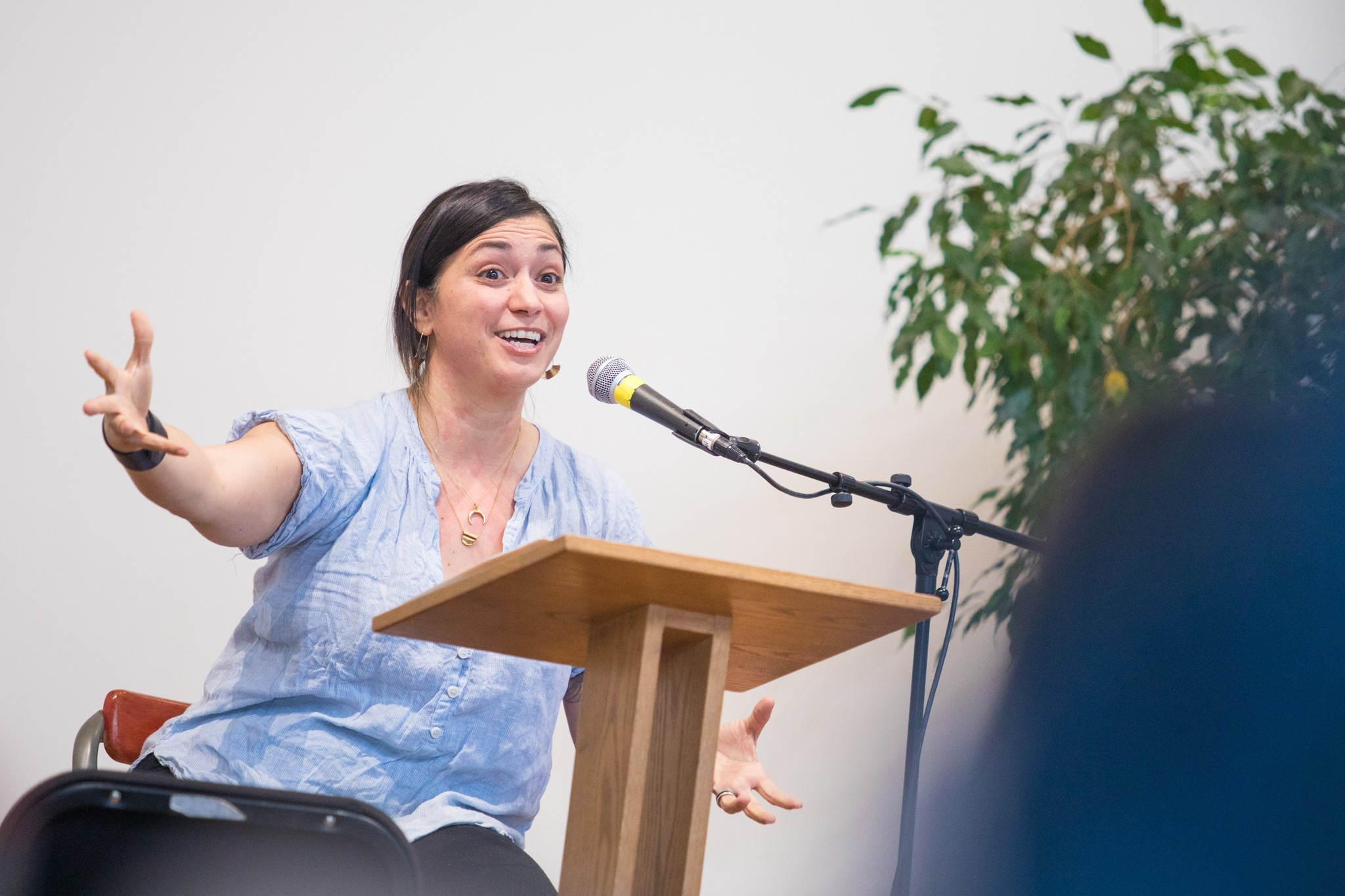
413, 399, 521, 546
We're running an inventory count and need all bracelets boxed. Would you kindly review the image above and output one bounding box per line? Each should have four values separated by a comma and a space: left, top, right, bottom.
102, 407, 168, 471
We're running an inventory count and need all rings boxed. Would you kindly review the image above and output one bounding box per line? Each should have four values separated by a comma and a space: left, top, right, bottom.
716, 789, 736, 808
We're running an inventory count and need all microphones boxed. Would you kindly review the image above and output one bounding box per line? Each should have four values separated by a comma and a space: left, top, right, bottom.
586, 355, 747, 463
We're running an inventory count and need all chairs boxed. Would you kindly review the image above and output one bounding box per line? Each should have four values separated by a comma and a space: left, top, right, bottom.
0, 768, 421, 896
69, 690, 190, 776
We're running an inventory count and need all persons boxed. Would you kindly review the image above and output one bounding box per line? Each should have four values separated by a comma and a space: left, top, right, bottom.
83, 178, 802, 896
